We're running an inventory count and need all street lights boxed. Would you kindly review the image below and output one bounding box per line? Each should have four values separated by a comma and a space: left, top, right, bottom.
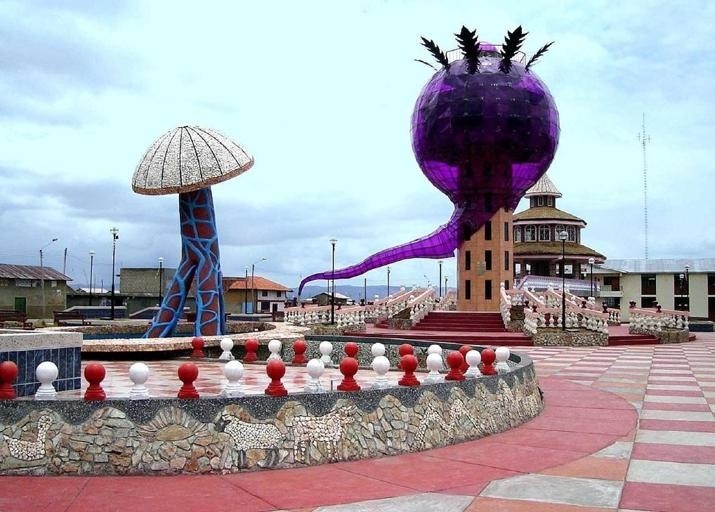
438, 260, 448, 296
245, 258, 266, 313
558, 231, 569, 331
110, 228, 119, 320
385, 266, 391, 296
158, 258, 163, 307
364, 278, 366, 300
589, 258, 595, 296
63, 248, 68, 275
329, 238, 338, 325
40, 238, 57, 318
679, 273, 684, 312
89, 251, 94, 305
